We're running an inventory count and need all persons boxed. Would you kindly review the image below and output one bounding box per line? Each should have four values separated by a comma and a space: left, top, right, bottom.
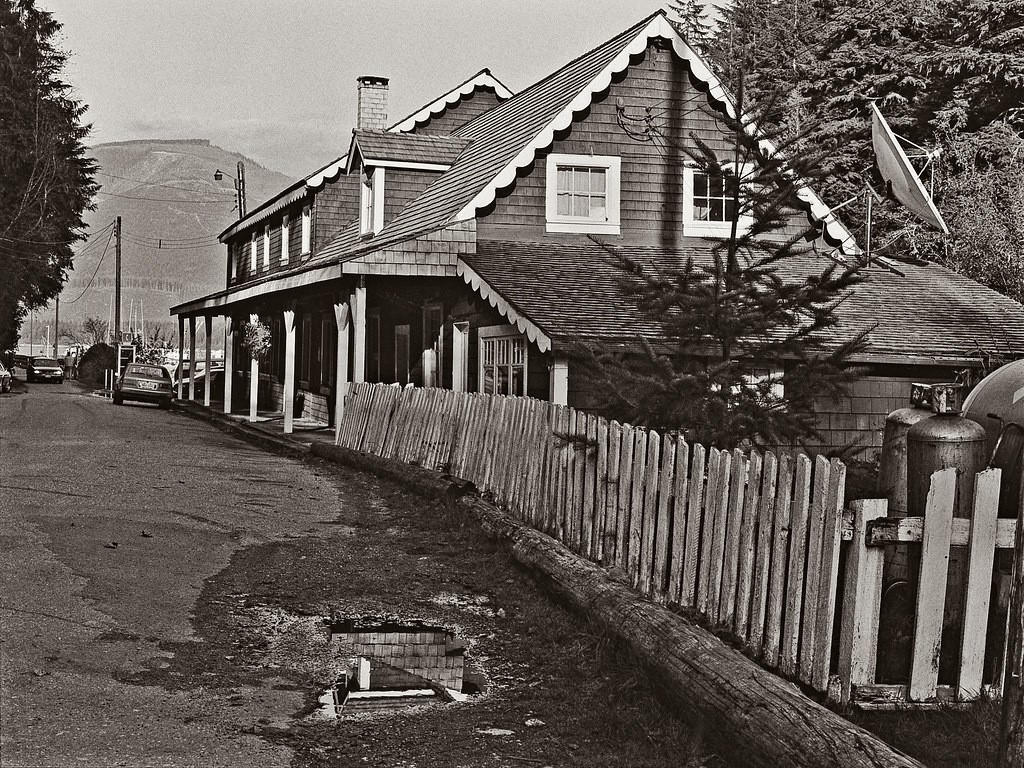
64, 351, 74, 382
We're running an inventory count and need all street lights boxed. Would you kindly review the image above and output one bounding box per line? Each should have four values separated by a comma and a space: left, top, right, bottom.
214, 168, 242, 219
46, 326, 49, 357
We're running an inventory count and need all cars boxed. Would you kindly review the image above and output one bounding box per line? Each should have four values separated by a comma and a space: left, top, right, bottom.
173, 367, 225, 398
113, 364, 174, 409
27, 357, 64, 384
0, 362, 11, 392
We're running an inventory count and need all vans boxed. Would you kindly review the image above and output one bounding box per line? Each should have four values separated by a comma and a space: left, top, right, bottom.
174, 358, 225, 383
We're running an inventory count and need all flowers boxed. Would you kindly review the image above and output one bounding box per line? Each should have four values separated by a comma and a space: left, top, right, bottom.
240, 322, 273, 361
